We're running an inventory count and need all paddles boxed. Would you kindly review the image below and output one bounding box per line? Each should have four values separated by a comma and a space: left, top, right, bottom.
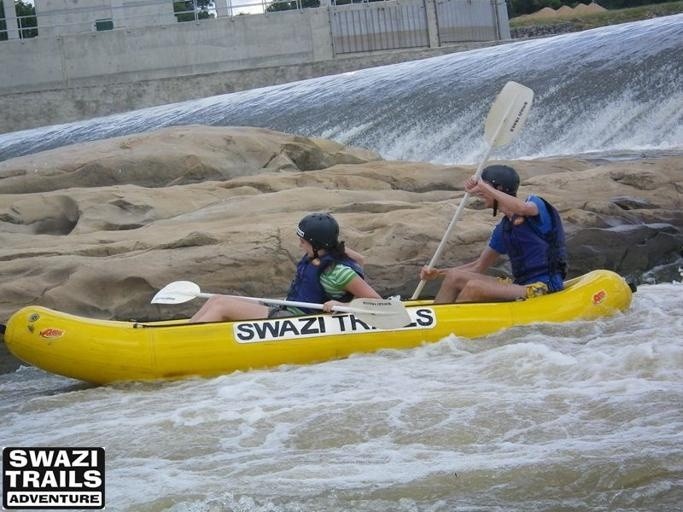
413, 82, 533, 299
150, 279, 411, 330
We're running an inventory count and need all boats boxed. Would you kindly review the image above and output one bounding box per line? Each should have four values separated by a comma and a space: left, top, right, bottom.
1, 267, 639, 390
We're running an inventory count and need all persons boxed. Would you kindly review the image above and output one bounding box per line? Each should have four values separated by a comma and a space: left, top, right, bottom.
189, 213, 383, 324
421, 165, 566, 305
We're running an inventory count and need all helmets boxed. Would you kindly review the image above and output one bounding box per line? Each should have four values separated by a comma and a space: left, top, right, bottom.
297, 213, 339, 248
482, 165, 519, 195
